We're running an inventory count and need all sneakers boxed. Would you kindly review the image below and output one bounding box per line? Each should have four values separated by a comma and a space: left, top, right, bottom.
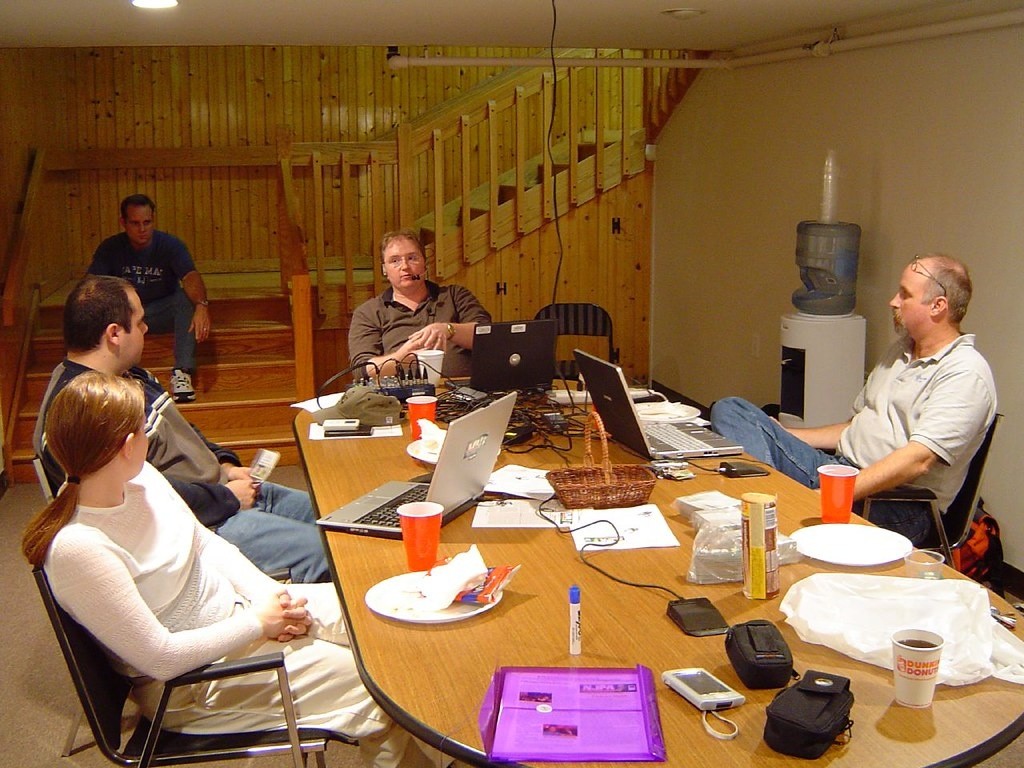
169, 367, 195, 402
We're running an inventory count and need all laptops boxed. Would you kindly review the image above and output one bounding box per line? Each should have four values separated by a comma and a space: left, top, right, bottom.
315, 391, 517, 539
444, 318, 558, 394
572, 348, 743, 462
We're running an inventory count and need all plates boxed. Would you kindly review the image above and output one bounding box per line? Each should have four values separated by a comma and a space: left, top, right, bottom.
407, 439, 501, 464
364, 570, 503, 624
789, 524, 913, 566
635, 402, 701, 424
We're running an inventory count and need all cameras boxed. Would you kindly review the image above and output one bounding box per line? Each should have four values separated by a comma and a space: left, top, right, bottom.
661, 667, 745, 712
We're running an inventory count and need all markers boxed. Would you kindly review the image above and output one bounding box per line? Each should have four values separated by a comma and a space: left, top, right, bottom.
570, 585, 582, 656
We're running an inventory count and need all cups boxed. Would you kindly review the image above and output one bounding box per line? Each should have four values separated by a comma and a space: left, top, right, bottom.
891, 628, 944, 709
406, 396, 438, 440
820, 149, 836, 224
904, 549, 945, 580
396, 502, 444, 571
816, 464, 860, 524
417, 349, 445, 388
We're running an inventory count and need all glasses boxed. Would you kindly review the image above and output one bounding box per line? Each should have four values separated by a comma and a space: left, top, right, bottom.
912, 253, 947, 297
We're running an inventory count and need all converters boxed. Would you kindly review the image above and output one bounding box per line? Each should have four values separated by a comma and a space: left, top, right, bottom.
542, 412, 568, 431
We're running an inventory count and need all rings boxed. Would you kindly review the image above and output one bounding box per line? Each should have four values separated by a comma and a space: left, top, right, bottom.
203, 327, 208, 332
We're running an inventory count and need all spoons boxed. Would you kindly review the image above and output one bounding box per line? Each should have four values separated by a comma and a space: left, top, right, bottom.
990, 606, 1015, 627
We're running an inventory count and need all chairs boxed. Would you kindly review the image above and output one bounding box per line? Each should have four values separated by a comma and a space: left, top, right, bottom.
531, 302, 621, 382
30, 560, 361, 768
32, 449, 293, 586
862, 413, 1004, 569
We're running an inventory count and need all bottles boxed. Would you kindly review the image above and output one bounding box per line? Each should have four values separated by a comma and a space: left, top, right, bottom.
791, 219, 862, 316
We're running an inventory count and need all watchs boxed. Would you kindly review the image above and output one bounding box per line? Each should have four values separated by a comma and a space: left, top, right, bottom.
193, 299, 211, 306
446, 322, 456, 340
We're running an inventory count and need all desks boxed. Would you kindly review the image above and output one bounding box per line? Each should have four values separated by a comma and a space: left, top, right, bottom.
291, 375, 1024, 768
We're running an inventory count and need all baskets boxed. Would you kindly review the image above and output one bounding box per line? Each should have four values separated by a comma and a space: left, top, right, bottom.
545, 411, 657, 510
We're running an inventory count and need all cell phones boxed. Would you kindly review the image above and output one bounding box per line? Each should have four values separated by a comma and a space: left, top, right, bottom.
323, 419, 360, 431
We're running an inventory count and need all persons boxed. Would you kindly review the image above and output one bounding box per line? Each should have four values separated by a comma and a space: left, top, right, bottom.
347, 228, 491, 381
711, 252, 997, 546
43, 273, 336, 584
87, 195, 211, 405
24, 370, 475, 767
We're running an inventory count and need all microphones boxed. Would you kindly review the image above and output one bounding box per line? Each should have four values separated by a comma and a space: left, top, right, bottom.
411, 268, 428, 279
